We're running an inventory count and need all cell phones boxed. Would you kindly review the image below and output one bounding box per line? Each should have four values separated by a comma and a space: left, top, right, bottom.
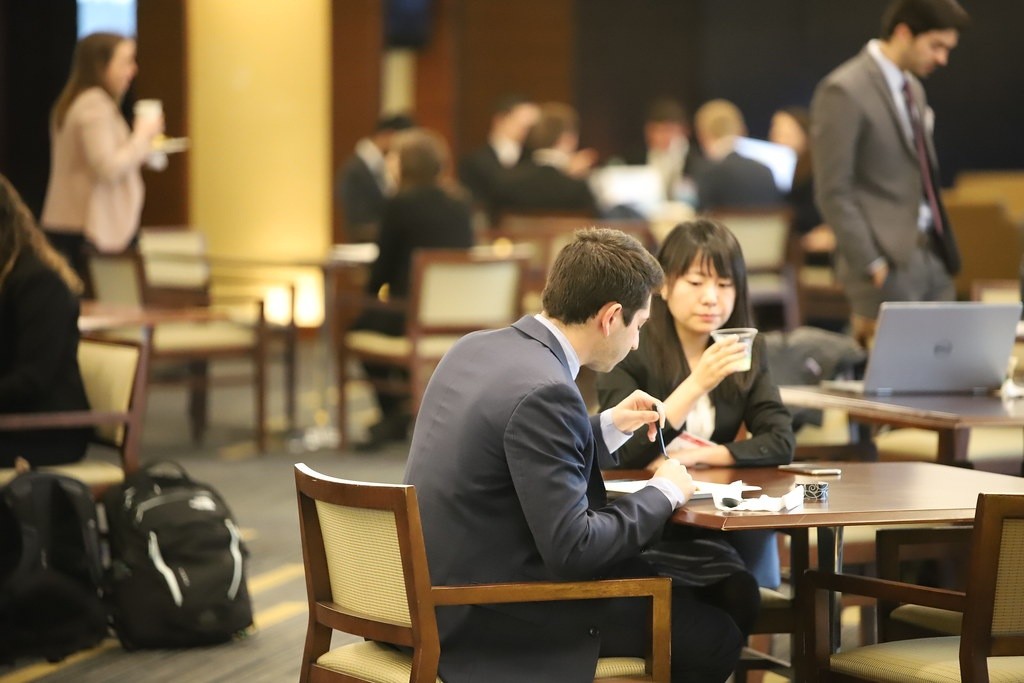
778, 464, 841, 474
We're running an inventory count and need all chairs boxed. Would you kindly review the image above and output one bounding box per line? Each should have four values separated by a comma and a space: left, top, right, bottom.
0, 207, 1024, 683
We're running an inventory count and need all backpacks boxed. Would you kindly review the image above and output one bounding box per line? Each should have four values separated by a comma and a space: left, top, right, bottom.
0, 471, 107, 674
102, 458, 252, 649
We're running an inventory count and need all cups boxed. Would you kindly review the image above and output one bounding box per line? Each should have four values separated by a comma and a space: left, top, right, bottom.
710, 328, 758, 371
134, 100, 163, 138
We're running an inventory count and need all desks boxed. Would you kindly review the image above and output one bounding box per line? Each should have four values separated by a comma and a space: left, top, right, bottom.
778, 386, 1024, 467
196, 241, 380, 449
602, 462, 1024, 683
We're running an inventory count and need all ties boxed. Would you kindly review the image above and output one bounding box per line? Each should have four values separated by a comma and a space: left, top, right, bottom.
902, 78, 944, 239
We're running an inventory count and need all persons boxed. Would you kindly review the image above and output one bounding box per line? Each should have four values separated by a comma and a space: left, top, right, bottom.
811, 1, 963, 344
342, 99, 807, 452
405, 225, 761, 683
39, 32, 163, 298
1, 177, 98, 469
594, 218, 794, 471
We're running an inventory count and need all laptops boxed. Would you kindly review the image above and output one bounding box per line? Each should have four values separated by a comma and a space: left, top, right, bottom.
821, 303, 1023, 396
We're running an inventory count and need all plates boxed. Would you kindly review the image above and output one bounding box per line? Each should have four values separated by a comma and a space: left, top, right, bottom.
604, 479, 726, 498
